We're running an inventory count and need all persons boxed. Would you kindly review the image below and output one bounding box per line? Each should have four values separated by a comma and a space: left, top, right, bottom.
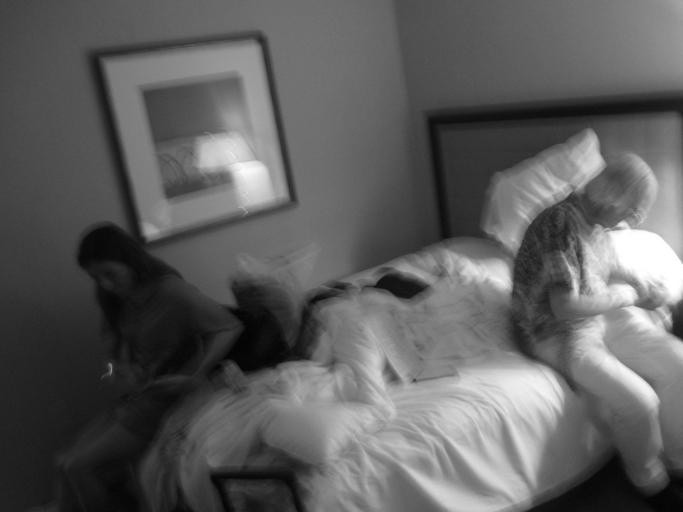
42, 221, 244, 511
511, 152, 683, 511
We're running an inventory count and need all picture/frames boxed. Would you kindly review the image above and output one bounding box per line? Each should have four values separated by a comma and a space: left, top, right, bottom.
89, 29, 300, 247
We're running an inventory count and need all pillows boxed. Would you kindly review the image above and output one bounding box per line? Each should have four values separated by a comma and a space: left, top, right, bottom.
475, 126, 681, 309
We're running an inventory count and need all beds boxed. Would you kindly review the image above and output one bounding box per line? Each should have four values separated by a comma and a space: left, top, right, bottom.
121, 92, 681, 512
